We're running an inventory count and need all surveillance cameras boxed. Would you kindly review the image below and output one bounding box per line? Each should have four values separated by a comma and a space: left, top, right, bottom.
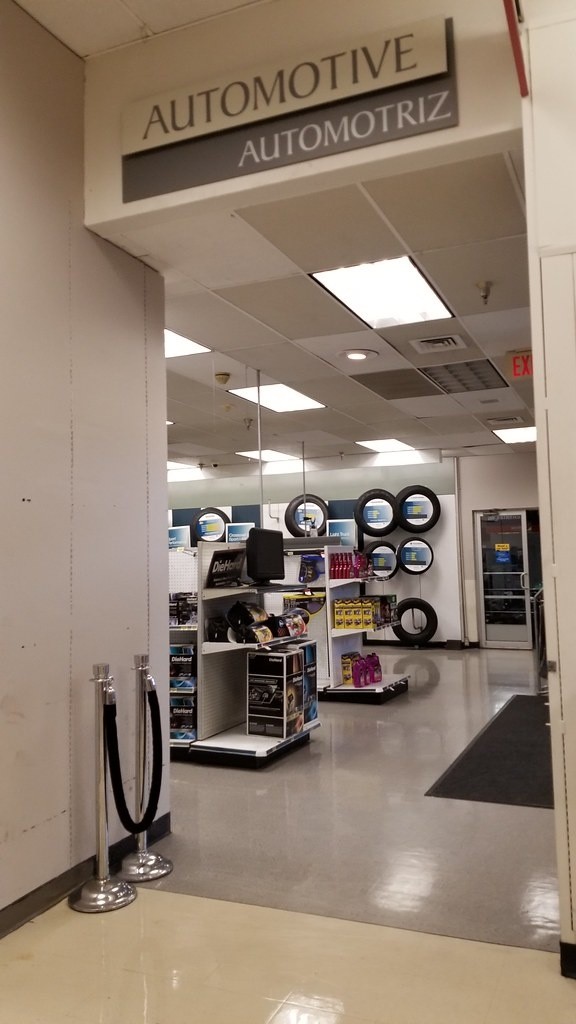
478, 281, 493, 299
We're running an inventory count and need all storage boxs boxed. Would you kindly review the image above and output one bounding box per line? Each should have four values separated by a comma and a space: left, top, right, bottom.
246, 639, 318, 740
333, 594, 397, 630
168, 643, 197, 742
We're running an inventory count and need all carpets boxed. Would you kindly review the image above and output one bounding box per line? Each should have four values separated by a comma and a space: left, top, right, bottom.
424, 694, 554, 810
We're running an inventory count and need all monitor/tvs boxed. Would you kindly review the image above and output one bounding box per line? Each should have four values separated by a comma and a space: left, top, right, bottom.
168, 526, 191, 549
246, 527, 285, 586
225, 523, 256, 544
326, 519, 358, 550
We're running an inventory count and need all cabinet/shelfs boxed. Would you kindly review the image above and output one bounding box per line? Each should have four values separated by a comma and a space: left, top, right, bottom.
263, 544, 410, 705
166, 540, 322, 770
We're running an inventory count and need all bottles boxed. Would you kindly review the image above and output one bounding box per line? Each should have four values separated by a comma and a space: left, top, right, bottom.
330, 553, 354, 579
353, 653, 382, 688
